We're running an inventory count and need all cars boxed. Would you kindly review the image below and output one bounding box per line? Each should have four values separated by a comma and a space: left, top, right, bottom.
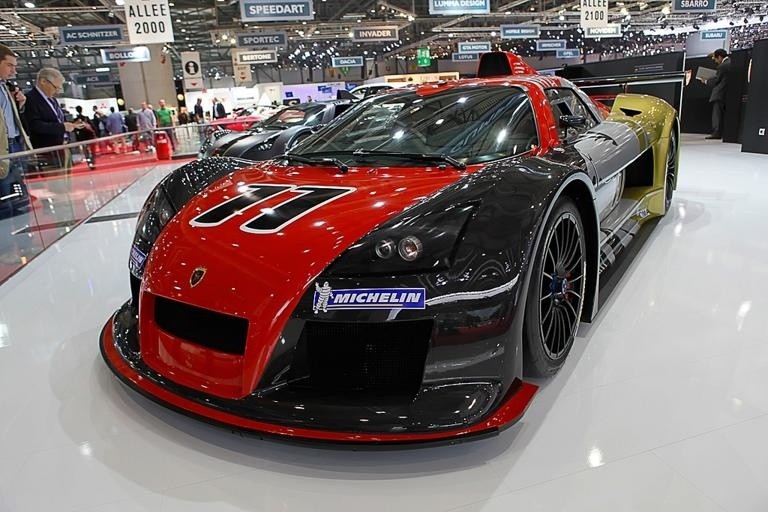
0, 160, 30, 219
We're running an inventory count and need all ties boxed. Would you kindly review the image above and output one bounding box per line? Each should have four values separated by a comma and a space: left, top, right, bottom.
47, 97, 64, 123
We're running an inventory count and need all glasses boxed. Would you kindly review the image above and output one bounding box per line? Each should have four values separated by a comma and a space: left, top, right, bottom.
47, 79, 63, 91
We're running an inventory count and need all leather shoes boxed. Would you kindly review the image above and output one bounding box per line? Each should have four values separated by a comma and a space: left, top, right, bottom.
704, 133, 722, 139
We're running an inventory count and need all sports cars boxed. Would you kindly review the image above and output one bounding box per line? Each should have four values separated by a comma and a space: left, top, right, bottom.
98, 50, 681, 452
200, 82, 395, 164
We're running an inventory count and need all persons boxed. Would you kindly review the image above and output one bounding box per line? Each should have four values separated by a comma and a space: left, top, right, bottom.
0, 44, 226, 196
307, 96, 312, 102
700, 49, 732, 139
